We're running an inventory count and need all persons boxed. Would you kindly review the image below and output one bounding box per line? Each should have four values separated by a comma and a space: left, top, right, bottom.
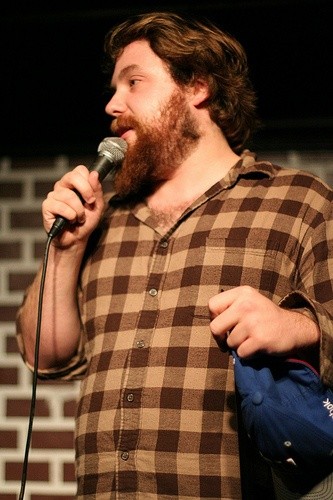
17, 12, 333, 500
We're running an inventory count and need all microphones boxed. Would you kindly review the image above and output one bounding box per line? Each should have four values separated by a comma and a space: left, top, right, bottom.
48, 136, 127, 239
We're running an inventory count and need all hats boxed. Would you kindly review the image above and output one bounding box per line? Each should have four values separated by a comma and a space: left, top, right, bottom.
229, 345, 333, 494
